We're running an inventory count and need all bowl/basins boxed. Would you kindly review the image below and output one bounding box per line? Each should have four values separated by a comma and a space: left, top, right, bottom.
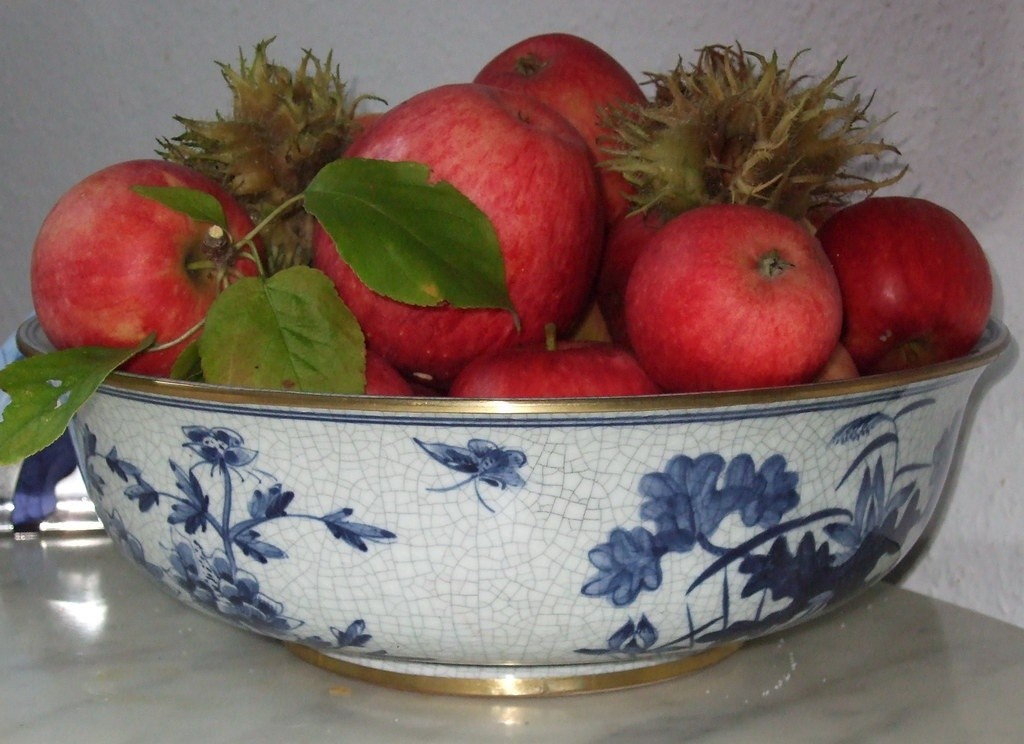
16, 307, 1011, 697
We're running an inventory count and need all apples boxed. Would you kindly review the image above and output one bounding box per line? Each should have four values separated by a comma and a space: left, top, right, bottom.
30, 34, 994, 395
